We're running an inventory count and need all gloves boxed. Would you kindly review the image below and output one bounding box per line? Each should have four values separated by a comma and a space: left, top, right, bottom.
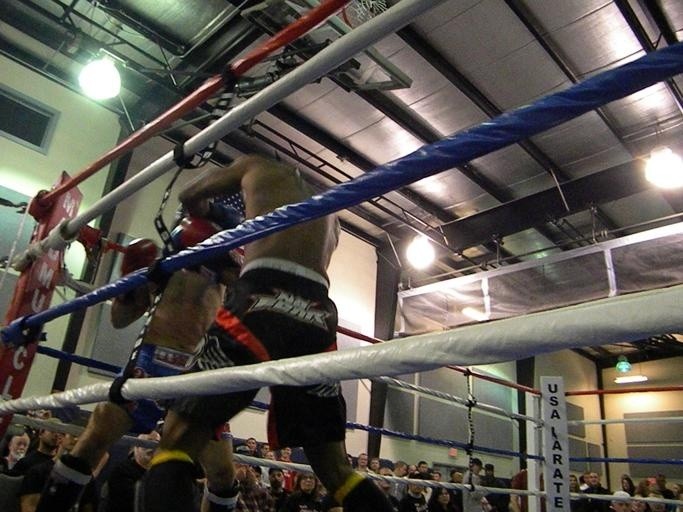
161, 193, 244, 268
120, 238, 157, 276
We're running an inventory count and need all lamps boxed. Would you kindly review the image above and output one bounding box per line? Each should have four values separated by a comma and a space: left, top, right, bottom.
614, 363, 648, 384
616, 342, 631, 373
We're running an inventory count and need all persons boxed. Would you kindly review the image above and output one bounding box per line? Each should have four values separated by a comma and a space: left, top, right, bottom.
33, 205, 244, 512
136, 148, 397, 512
